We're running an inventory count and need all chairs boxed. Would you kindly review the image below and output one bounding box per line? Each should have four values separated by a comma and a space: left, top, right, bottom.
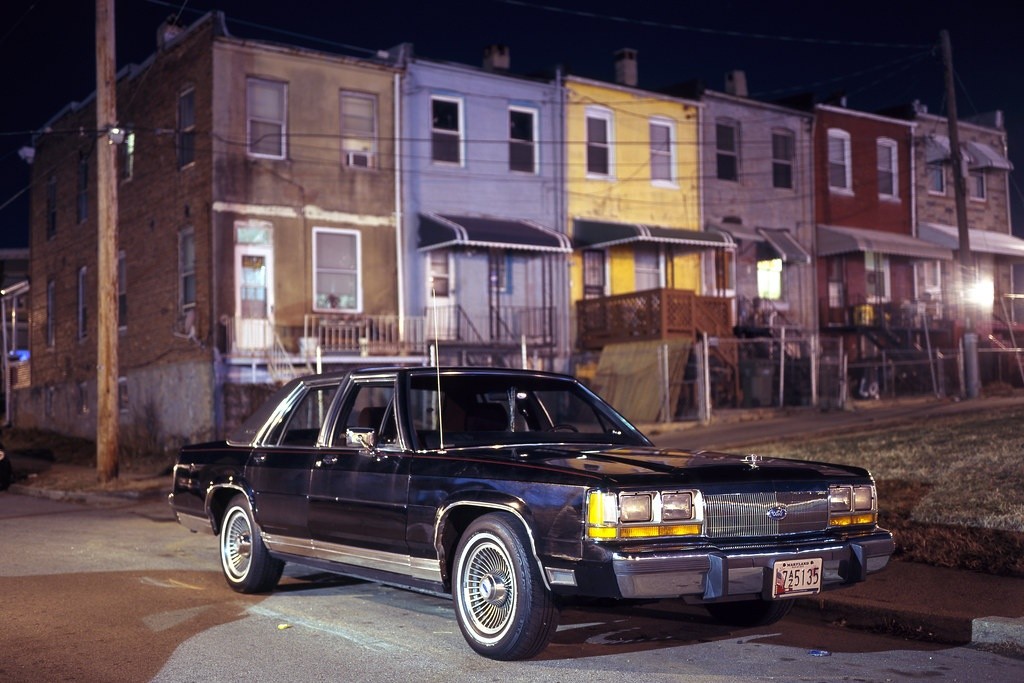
465, 400, 508, 432
359, 405, 397, 435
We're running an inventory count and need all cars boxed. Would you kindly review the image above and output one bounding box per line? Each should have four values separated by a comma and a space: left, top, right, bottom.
169, 366, 895, 662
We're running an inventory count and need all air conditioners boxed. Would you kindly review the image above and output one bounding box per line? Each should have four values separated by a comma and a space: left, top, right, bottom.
344, 151, 369, 168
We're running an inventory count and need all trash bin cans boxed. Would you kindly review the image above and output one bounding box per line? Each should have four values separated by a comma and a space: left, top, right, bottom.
741, 354, 771, 408
774, 369, 804, 406
